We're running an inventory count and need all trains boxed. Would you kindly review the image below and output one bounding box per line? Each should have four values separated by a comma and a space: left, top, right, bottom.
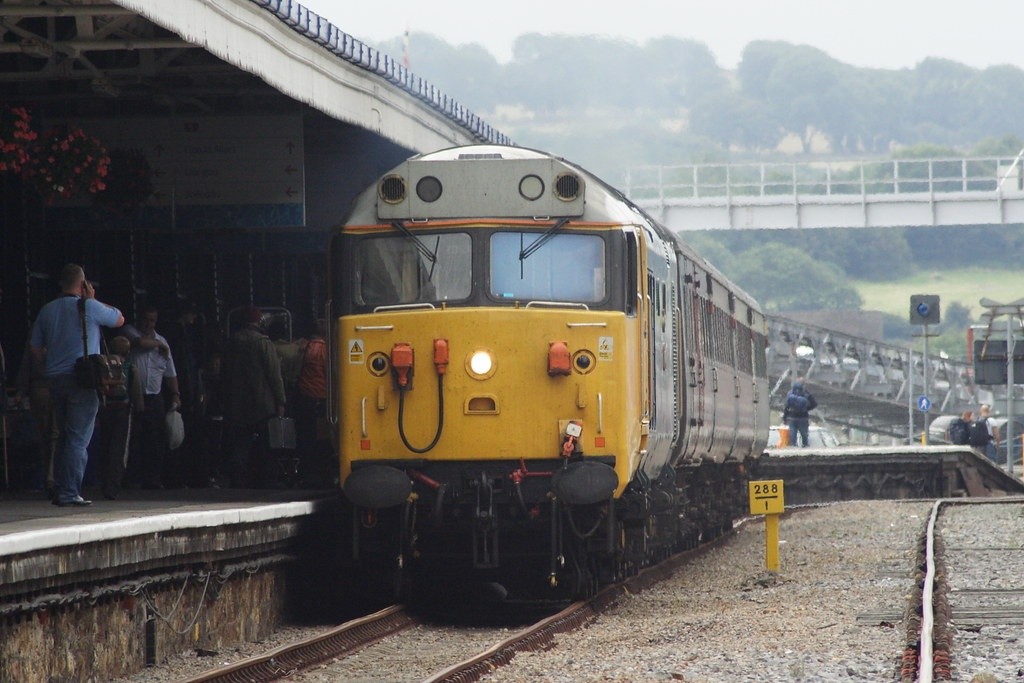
325, 145, 771, 608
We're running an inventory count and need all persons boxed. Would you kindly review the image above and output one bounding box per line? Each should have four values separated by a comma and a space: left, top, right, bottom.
782, 377, 817, 446
961, 410, 977, 443
30, 264, 125, 506
117, 306, 181, 493
96, 336, 142, 502
978, 405, 999, 462
13, 324, 54, 497
170, 301, 326, 488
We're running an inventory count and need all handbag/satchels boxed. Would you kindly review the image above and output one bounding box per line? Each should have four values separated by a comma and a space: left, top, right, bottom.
165, 403, 183, 448
75, 353, 128, 400
286, 339, 311, 386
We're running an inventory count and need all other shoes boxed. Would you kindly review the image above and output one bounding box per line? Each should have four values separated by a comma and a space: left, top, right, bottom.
53, 492, 92, 507
101, 482, 127, 501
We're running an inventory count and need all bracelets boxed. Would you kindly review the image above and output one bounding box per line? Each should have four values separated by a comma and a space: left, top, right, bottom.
172, 392, 181, 395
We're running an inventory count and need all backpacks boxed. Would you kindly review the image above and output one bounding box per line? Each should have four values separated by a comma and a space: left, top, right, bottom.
948, 417, 969, 445
968, 416, 994, 448
786, 393, 808, 413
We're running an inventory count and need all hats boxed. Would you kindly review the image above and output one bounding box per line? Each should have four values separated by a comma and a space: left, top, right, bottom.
247, 308, 266, 324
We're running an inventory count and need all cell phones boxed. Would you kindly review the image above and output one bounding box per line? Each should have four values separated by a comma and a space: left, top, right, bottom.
83, 280, 87, 290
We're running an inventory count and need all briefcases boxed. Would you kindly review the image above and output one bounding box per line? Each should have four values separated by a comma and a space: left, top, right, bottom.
268, 417, 296, 451
